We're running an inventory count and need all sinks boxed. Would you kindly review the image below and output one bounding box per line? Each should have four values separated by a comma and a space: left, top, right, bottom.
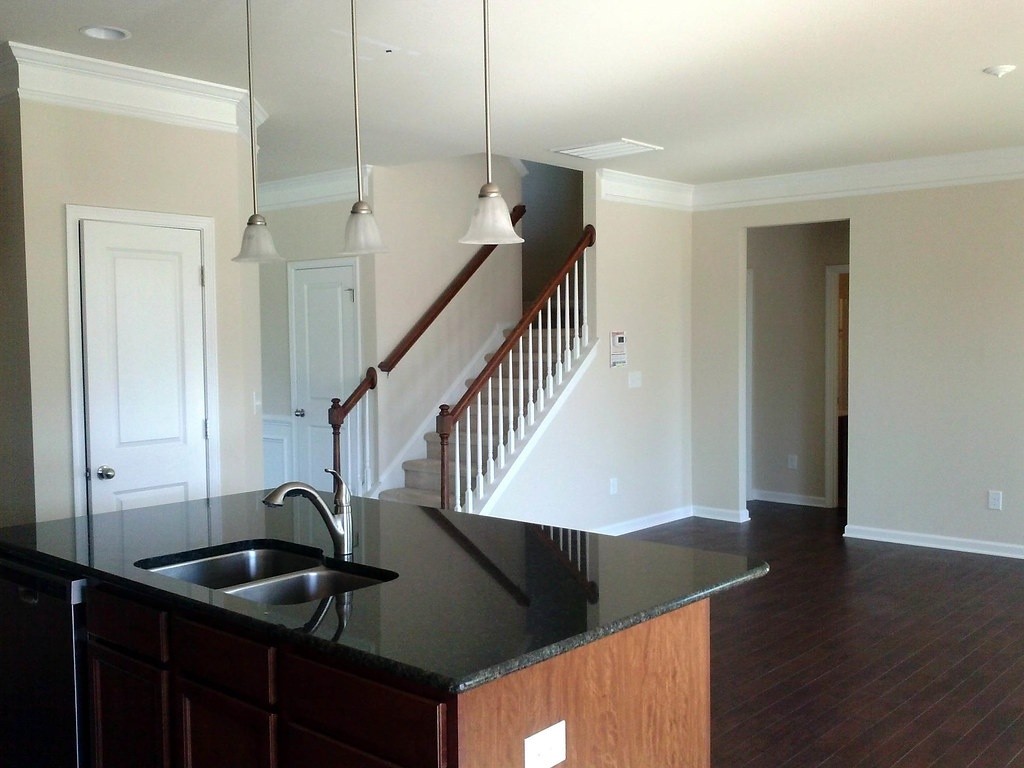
132, 537, 328, 591
222, 560, 401, 608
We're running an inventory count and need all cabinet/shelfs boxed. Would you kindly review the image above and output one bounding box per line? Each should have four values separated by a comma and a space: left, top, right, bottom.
0, 550, 457, 768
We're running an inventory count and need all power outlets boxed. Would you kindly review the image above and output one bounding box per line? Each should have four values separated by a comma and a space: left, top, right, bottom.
987, 490, 1003, 510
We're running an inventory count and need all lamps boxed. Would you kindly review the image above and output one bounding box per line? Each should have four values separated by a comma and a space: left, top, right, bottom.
231, 0, 286, 264
456, 0, 525, 245
334, 1, 391, 256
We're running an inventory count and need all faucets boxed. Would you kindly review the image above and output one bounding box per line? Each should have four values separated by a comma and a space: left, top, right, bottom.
273, 590, 355, 644
259, 466, 356, 564
